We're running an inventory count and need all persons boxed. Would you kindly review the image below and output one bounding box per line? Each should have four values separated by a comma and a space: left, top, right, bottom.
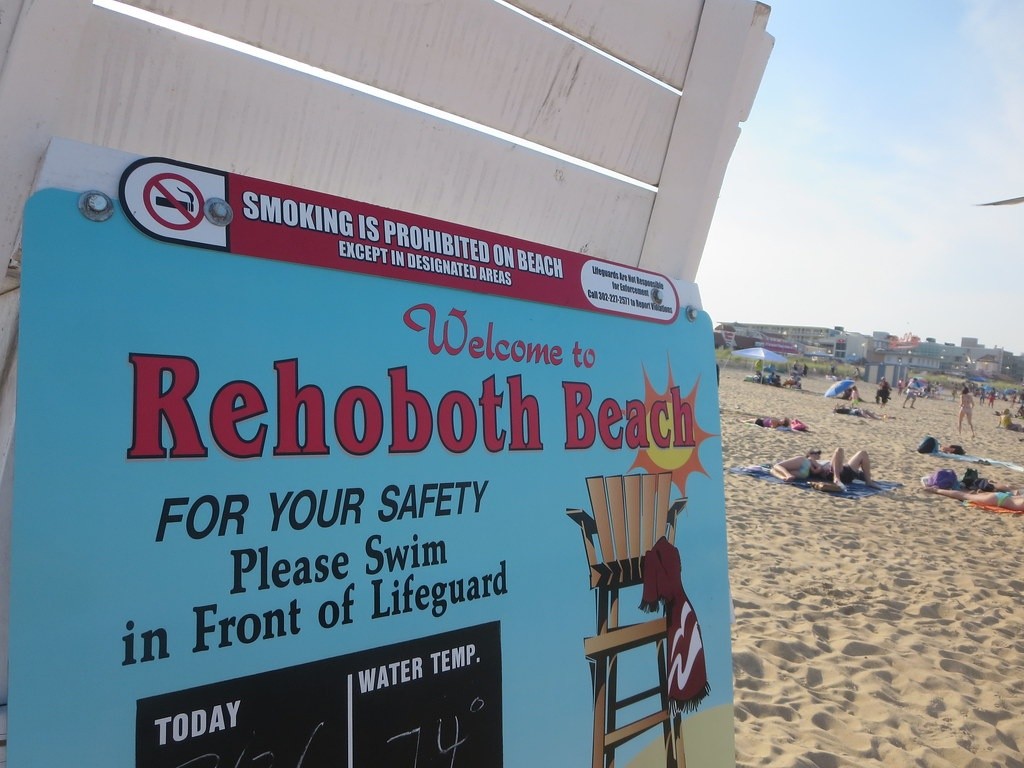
754, 359, 1024, 422
958, 387, 975, 438
995, 409, 1024, 432
736, 416, 791, 428
923, 485, 1024, 511
769, 446, 883, 492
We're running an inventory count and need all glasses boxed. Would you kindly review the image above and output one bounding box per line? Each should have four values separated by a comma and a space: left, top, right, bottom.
810, 450, 821, 455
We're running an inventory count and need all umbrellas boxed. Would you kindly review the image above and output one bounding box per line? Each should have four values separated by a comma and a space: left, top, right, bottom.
731, 347, 789, 384
825, 380, 854, 401
